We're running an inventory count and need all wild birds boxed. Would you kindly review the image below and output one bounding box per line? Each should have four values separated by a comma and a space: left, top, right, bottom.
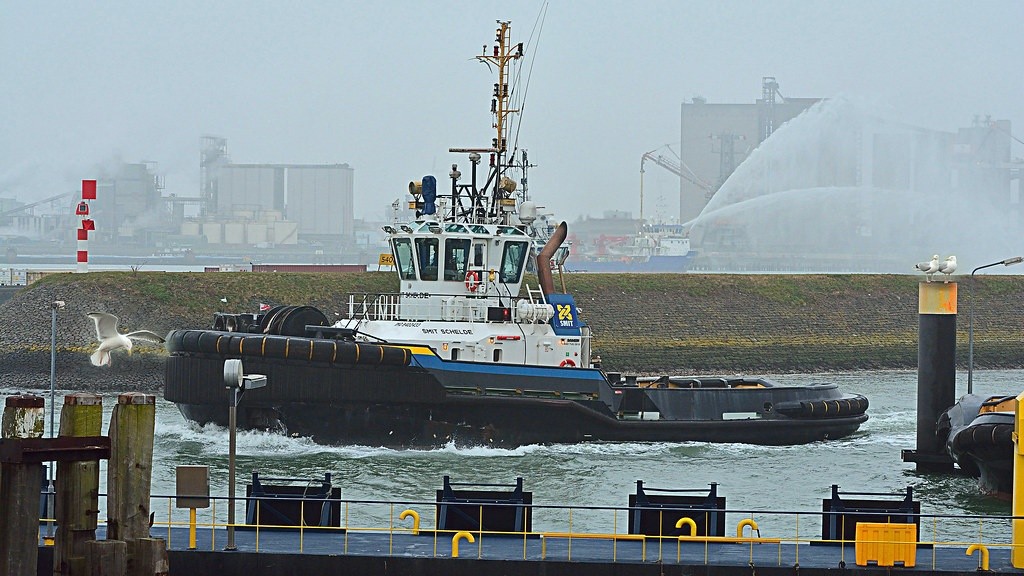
86, 312, 166, 368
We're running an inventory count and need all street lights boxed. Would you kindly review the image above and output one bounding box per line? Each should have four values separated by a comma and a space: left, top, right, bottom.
966, 255, 1024, 393
45, 300, 66, 545
220, 357, 267, 551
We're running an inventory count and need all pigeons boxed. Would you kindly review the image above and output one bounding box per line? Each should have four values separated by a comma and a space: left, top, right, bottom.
913, 254, 939, 283
939, 256, 958, 284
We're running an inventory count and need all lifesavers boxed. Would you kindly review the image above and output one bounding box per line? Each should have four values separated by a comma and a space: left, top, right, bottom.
465, 272, 478, 291
560, 359, 575, 367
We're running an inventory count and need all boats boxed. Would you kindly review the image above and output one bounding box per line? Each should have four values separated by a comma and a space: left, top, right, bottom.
162, 17, 872, 449
